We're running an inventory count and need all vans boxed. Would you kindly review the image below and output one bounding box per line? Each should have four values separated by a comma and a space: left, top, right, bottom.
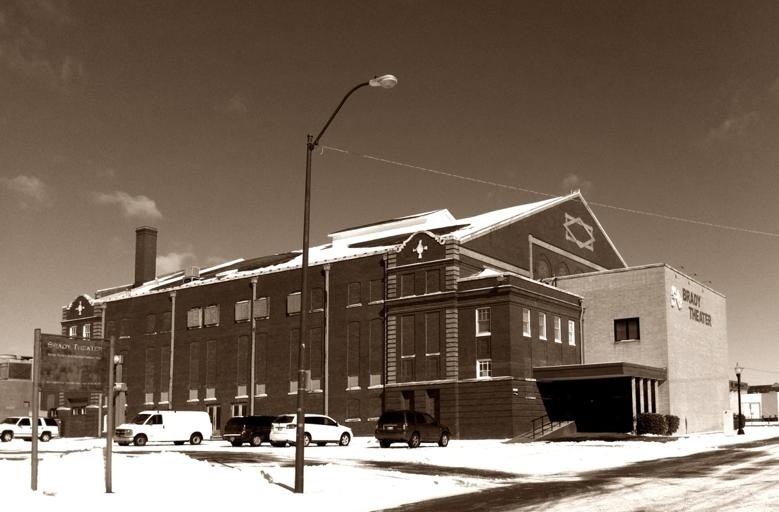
114, 410, 214, 446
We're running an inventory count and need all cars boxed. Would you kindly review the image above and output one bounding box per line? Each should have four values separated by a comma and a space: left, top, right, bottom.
222, 409, 451, 447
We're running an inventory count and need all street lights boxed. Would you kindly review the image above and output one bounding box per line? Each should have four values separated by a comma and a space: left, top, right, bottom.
294, 73, 398, 493
734, 362, 744, 435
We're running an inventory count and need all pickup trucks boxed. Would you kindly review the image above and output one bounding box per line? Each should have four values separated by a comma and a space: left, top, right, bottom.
0, 415, 60, 442
